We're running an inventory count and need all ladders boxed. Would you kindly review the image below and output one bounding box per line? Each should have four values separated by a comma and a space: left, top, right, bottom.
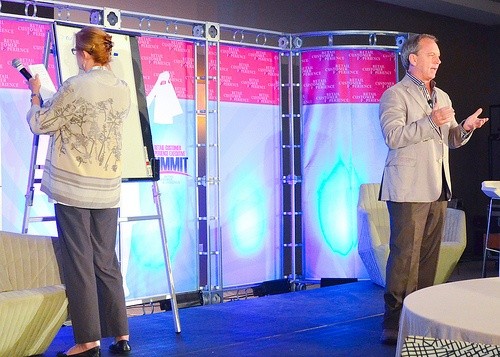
20, 30, 181, 336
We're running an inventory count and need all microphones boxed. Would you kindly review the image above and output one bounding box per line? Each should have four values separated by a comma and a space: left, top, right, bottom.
12, 59, 32, 81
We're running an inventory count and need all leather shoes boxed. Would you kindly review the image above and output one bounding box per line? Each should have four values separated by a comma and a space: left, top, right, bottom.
108, 339, 131, 353
56, 346, 103, 357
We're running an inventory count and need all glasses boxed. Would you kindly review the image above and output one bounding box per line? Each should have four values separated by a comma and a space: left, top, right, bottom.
71, 47, 91, 55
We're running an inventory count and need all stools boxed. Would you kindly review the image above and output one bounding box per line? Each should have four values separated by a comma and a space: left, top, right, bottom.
481, 181, 500, 280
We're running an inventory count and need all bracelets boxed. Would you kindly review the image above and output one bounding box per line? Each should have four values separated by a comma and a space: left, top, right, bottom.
31, 93, 41, 100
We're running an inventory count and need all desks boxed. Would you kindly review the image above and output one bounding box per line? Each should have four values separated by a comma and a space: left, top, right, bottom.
395, 277, 500, 357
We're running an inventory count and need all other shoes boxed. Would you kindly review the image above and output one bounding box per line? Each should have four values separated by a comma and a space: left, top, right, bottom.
380, 328, 398, 344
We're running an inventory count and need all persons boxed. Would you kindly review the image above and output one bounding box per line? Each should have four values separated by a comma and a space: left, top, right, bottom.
28, 27, 133, 357
375, 34, 489, 346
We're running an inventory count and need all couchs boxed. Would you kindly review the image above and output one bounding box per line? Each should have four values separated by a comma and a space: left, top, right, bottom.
0, 231, 70, 357
358, 184, 467, 288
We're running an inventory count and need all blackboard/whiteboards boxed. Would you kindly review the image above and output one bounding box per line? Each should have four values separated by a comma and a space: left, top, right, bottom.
50, 20, 160, 182
